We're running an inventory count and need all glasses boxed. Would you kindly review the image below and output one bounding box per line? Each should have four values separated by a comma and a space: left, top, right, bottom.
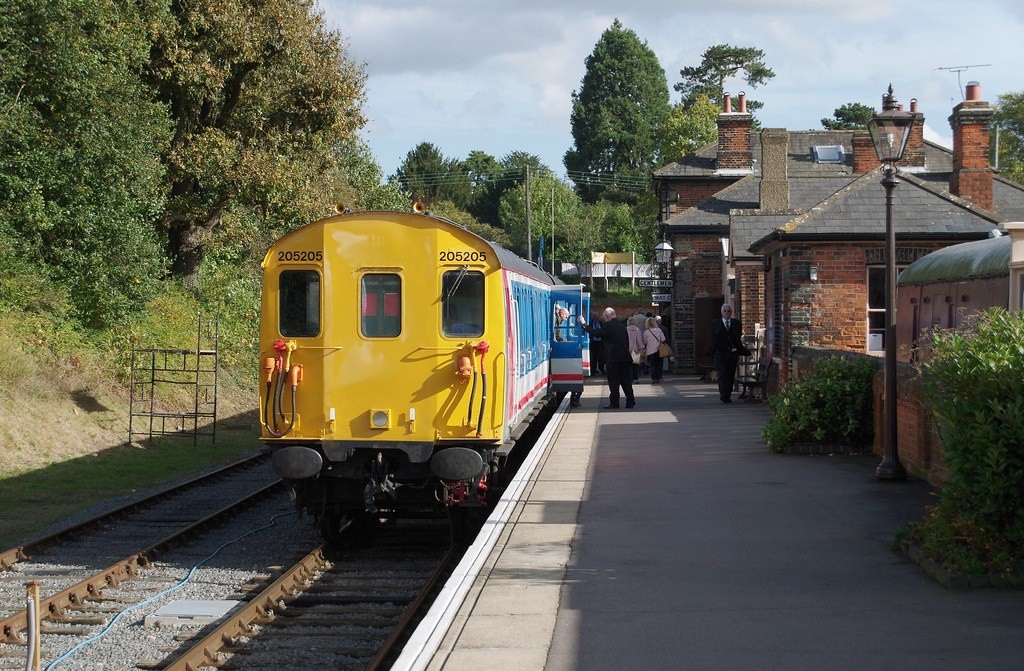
723, 309, 731, 312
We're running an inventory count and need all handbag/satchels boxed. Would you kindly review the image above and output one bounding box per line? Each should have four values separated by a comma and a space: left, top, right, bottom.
658, 343, 673, 357
630, 350, 641, 364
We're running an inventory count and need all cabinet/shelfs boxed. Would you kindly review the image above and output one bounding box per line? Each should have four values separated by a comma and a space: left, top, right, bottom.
737, 331, 759, 399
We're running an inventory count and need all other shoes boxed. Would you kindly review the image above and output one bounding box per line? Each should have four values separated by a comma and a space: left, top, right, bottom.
570, 398, 582, 407
720, 395, 733, 403
643, 367, 649, 375
603, 404, 620, 409
625, 400, 636, 408
635, 379, 639, 384
651, 379, 660, 384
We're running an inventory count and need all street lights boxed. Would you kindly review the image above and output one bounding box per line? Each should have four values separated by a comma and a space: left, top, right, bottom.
867, 82, 916, 481
653, 233, 674, 320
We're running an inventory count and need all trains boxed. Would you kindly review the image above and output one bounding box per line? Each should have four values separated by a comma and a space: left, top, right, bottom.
259, 204, 592, 543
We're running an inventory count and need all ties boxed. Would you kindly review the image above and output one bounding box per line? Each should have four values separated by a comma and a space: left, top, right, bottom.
725, 320, 730, 331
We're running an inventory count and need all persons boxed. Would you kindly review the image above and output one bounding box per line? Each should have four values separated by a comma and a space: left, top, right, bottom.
577, 307, 637, 409
554, 304, 584, 408
705, 303, 742, 403
626, 308, 668, 384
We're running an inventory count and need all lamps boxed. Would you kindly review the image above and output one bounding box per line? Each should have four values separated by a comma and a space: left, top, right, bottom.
654, 232, 674, 265
809, 266, 818, 282
